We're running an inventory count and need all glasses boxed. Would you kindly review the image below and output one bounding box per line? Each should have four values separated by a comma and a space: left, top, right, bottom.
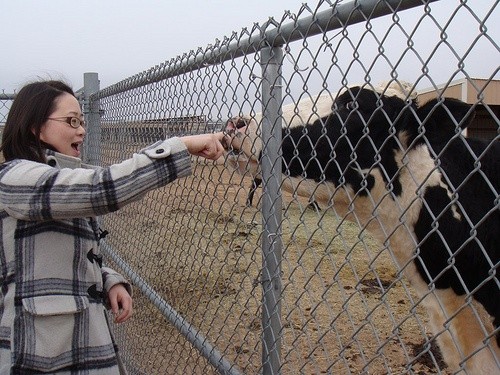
47, 116, 85, 129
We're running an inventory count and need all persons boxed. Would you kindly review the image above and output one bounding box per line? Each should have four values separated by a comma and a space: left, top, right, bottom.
0, 80, 233, 375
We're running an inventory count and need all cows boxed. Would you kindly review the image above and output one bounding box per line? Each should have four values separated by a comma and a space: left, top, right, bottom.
220, 80, 500, 375
